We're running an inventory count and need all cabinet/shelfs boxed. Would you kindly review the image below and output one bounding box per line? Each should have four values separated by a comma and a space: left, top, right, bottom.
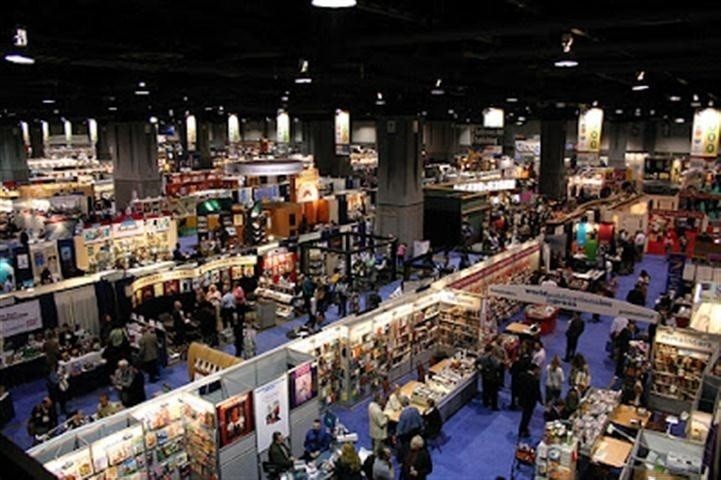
35, 392, 221, 479
642, 324, 713, 417
288, 288, 482, 415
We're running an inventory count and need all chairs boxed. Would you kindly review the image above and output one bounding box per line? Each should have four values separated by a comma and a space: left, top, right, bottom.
262, 441, 376, 480
420, 422, 444, 450
510, 446, 536, 479
